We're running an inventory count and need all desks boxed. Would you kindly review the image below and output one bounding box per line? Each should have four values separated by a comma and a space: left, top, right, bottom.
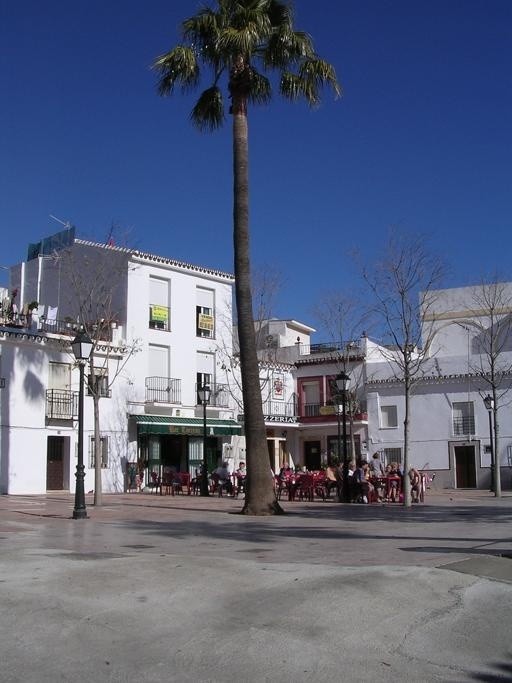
368, 477, 402, 504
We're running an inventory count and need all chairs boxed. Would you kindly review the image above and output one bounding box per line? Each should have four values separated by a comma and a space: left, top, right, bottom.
151, 469, 377, 505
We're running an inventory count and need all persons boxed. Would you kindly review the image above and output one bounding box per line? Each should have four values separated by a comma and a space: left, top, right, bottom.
325, 450, 435, 504
218, 461, 237, 495
237, 461, 247, 492
190, 467, 202, 494
281, 461, 289, 480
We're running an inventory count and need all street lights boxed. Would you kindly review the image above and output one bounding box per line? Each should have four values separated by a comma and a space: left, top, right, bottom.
336, 371, 350, 496
484, 394, 495, 486
70, 330, 93, 510
198, 386, 211, 491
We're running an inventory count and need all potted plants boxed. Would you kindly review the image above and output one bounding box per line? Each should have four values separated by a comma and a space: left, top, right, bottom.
4, 288, 118, 341
